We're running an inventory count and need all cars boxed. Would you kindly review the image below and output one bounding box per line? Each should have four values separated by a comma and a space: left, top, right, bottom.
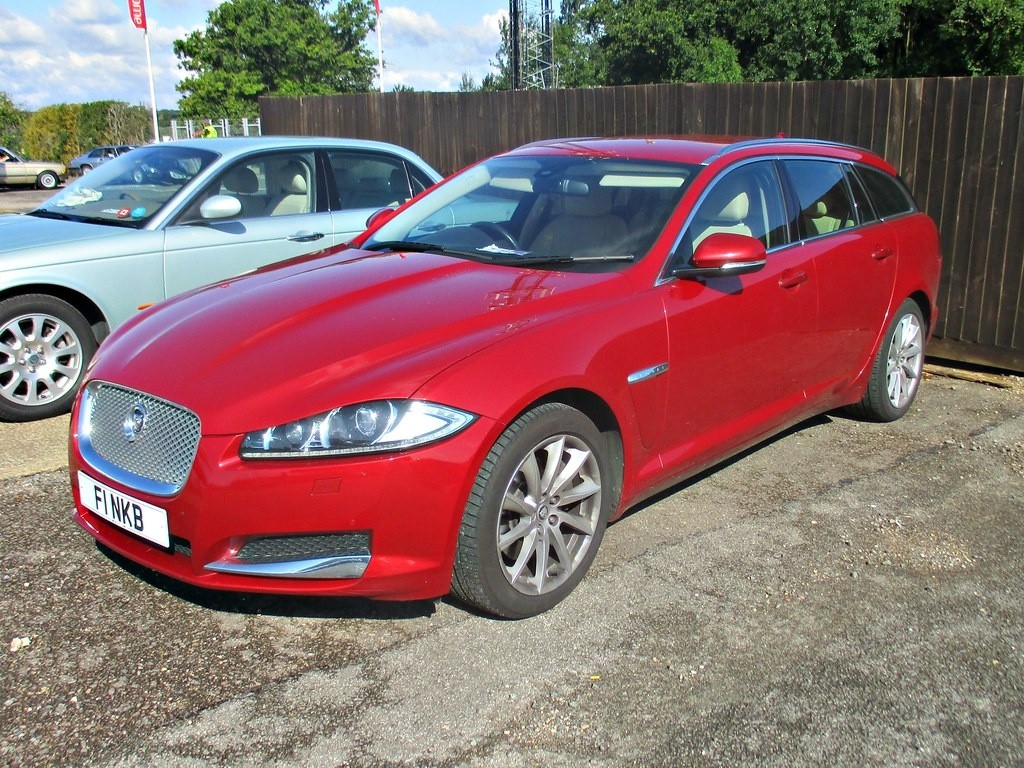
66, 133, 942, 623
69, 144, 141, 177
0, 136, 445, 425
0, 146, 67, 190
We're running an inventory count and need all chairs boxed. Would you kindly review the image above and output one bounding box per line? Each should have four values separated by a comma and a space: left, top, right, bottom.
351, 176, 389, 208
528, 176, 628, 258
223, 167, 266, 219
783, 184, 855, 244
381, 170, 411, 206
333, 168, 354, 203
690, 176, 753, 255
264, 164, 307, 215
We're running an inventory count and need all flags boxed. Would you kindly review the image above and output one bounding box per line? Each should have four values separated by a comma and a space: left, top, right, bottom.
128, 0, 148, 31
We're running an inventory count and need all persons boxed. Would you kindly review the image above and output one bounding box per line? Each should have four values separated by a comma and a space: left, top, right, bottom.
191, 118, 218, 138
